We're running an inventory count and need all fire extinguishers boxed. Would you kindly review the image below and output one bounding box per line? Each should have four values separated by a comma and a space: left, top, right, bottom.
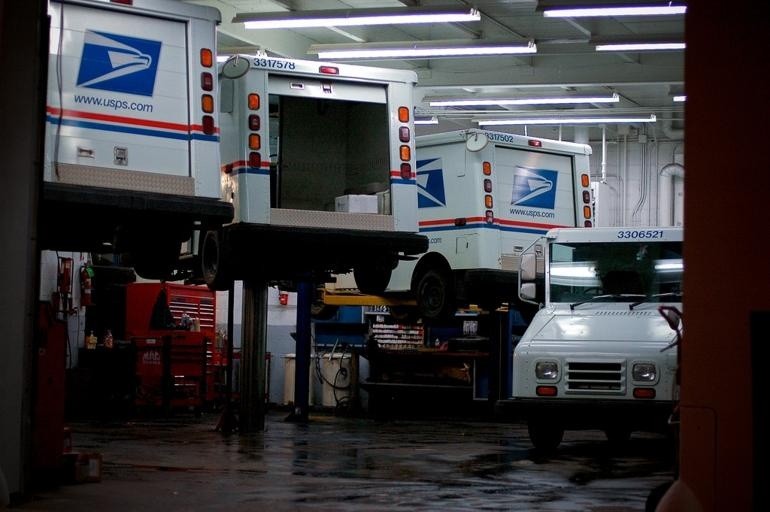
79, 263, 95, 306
59, 258, 72, 300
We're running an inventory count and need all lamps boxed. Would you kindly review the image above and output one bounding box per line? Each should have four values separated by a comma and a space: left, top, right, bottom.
536, 1, 687, 17
588, 40, 686, 54
306, 39, 538, 59
414, 117, 440, 125
423, 93, 620, 106
668, 92, 686, 102
473, 114, 657, 126
231, 7, 482, 29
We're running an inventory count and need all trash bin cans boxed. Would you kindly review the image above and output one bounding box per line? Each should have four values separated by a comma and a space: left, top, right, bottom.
280, 353, 353, 406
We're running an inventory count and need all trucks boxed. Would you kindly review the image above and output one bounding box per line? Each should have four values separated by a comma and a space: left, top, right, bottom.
509, 222, 684, 455
